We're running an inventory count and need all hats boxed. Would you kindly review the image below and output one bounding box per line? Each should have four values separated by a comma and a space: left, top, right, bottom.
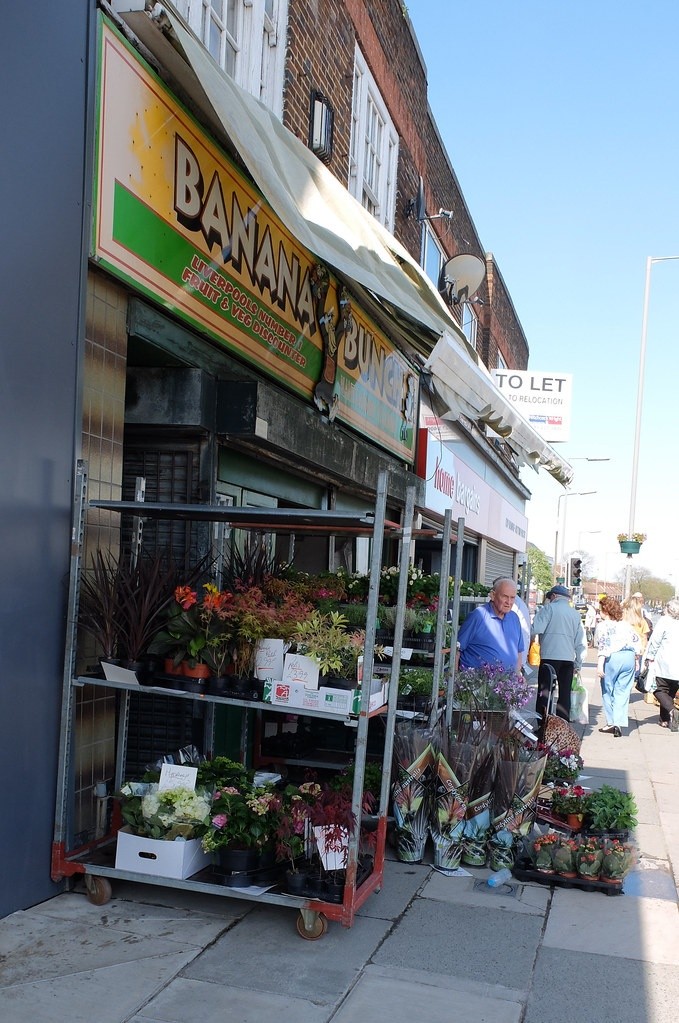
546, 586, 571, 599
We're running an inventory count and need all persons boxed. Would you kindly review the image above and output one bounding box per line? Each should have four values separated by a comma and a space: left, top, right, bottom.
619, 592, 650, 680
492, 577, 530, 676
454, 576, 524, 684
584, 602, 597, 648
593, 596, 642, 737
643, 599, 679, 732
529, 586, 588, 743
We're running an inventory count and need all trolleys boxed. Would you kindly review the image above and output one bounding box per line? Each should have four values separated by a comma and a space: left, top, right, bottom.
48, 468, 464, 941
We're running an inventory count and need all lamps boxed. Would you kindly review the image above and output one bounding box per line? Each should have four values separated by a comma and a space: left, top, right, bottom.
462, 296, 484, 304
418, 207, 454, 221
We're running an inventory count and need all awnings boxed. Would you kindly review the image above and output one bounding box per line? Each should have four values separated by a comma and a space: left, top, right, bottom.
160, 1, 577, 495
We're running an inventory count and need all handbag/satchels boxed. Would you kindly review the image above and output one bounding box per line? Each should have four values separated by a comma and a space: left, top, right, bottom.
570, 669, 589, 724
635, 661, 649, 693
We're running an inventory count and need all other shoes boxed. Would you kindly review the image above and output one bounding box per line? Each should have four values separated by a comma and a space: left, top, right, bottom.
658, 720, 668, 727
670, 708, 679, 732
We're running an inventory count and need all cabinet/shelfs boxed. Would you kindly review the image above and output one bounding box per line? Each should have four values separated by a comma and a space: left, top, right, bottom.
51, 463, 417, 942
250, 510, 470, 848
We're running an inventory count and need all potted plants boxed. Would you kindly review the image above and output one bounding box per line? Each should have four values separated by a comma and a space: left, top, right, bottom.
618, 530, 647, 554
92, 541, 388, 697
390, 702, 578, 872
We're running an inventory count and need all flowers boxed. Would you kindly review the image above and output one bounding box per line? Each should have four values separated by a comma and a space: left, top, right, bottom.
526, 746, 635, 879
114, 750, 382, 863
455, 661, 537, 714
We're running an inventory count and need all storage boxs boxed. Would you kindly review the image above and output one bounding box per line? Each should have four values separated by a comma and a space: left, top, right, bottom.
113, 819, 215, 880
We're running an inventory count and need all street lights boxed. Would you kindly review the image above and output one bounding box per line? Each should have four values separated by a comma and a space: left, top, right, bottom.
553, 489, 597, 584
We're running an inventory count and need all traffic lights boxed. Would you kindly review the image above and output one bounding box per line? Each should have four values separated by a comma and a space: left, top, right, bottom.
571, 558, 582, 586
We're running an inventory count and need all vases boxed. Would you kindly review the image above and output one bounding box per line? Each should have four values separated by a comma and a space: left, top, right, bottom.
220, 838, 374, 902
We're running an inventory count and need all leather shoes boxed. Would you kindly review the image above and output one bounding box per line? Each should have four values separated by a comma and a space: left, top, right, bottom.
613, 726, 621, 737
599, 726, 614, 733
